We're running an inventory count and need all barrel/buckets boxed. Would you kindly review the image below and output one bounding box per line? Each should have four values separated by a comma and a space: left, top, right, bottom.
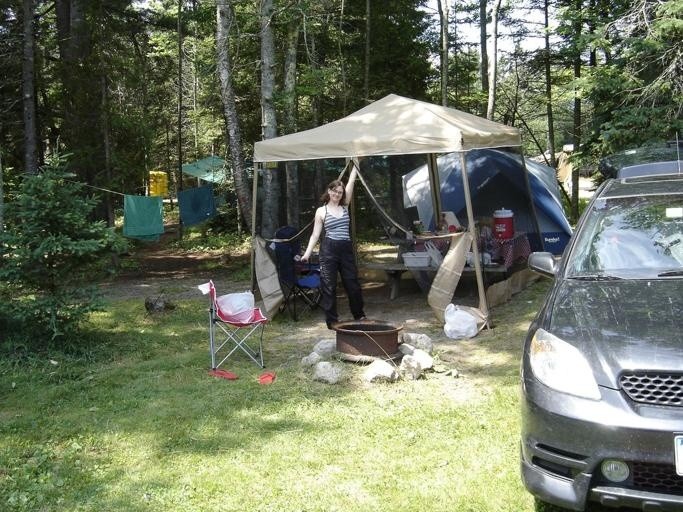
150, 170, 168, 199
493, 207, 514, 240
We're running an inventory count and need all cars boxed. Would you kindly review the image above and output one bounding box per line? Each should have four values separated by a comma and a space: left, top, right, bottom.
514, 140, 683, 511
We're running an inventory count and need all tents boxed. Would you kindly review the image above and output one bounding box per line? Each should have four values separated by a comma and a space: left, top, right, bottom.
398, 145, 574, 259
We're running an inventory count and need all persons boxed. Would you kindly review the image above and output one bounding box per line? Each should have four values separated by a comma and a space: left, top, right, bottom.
300, 157, 368, 333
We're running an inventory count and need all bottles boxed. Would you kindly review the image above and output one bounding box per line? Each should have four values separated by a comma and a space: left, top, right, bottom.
417, 221, 425, 234
438, 213, 448, 232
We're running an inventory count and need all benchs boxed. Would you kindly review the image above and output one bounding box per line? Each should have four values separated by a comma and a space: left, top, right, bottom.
365, 263, 510, 299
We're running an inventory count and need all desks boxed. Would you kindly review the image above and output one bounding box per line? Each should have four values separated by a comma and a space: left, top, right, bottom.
379, 231, 527, 299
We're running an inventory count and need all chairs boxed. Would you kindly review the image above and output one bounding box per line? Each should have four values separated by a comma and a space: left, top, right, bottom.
274, 226, 329, 321
207, 279, 267, 371
